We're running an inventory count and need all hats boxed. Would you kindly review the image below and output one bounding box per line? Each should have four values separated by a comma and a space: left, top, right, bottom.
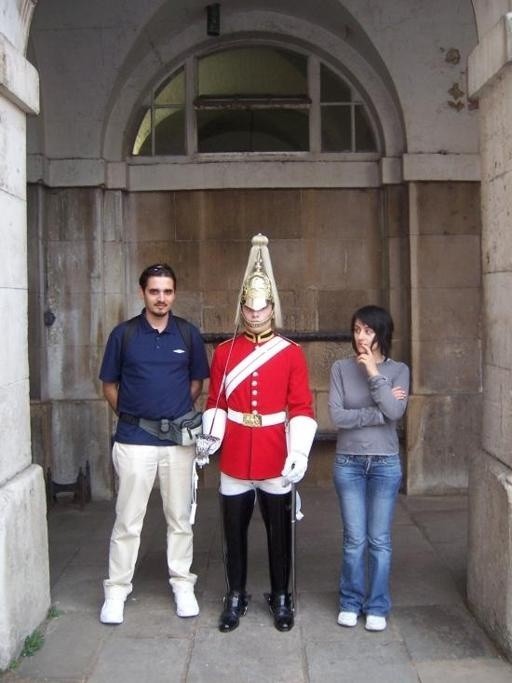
241, 270, 276, 310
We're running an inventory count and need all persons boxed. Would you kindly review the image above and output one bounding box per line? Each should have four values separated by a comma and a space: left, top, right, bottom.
98, 264, 211, 626
327, 305, 410, 633
196, 299, 319, 633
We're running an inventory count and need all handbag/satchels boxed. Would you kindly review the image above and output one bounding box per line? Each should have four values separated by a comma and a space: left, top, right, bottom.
159, 407, 202, 449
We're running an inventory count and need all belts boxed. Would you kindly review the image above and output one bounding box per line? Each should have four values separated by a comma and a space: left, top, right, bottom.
228, 409, 288, 430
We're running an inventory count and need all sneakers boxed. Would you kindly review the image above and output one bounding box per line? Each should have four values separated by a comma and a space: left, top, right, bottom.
170, 583, 200, 618
99, 593, 127, 625
364, 614, 387, 633
336, 610, 359, 626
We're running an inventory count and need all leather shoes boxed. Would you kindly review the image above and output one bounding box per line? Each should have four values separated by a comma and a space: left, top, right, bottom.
267, 593, 294, 632
218, 591, 247, 632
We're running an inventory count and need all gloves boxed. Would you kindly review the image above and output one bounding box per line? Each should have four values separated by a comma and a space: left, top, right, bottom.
281, 414, 319, 483
197, 406, 227, 466
